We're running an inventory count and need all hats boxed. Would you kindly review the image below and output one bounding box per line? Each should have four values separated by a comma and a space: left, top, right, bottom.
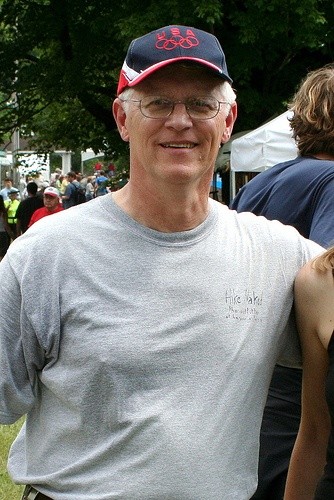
116, 24, 234, 95
44, 187, 59, 198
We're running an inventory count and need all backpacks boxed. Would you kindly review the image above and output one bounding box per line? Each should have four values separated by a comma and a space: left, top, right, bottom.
72, 184, 86, 203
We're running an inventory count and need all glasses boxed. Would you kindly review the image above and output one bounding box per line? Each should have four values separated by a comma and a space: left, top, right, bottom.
124, 94, 234, 119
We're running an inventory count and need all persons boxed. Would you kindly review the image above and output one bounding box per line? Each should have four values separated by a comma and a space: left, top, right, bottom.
0, 25, 328, 500
232, 68, 334, 500
282, 246, 334, 500
0, 167, 112, 260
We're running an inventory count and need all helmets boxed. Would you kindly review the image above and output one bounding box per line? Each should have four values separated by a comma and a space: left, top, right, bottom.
7, 188, 20, 196
